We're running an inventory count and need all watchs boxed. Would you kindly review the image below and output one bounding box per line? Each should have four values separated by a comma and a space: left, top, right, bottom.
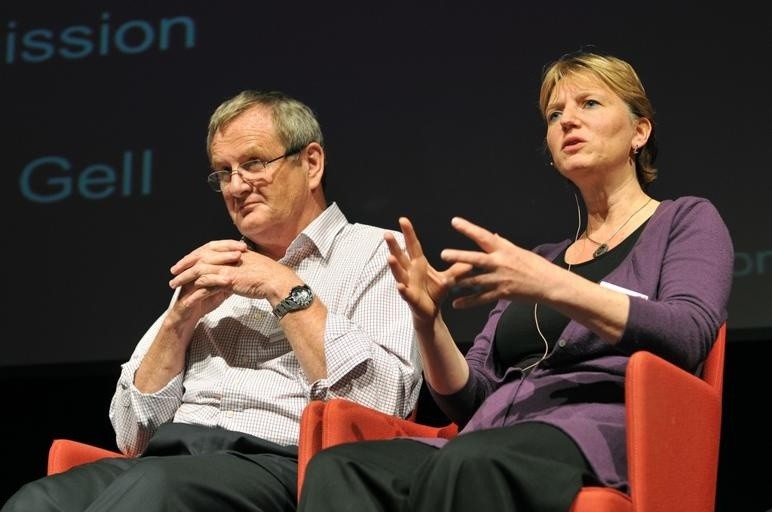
272, 281, 312, 321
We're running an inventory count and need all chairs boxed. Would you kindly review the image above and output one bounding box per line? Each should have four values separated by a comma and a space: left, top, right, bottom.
46, 399, 415, 512
321, 318, 726, 512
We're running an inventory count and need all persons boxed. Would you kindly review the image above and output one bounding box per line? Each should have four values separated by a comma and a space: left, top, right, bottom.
293, 48, 736, 511
1, 89, 432, 511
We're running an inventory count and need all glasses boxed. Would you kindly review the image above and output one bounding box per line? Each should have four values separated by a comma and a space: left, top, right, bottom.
204, 152, 288, 193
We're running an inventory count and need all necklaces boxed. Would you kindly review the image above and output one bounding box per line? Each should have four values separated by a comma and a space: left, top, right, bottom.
585, 198, 656, 258
